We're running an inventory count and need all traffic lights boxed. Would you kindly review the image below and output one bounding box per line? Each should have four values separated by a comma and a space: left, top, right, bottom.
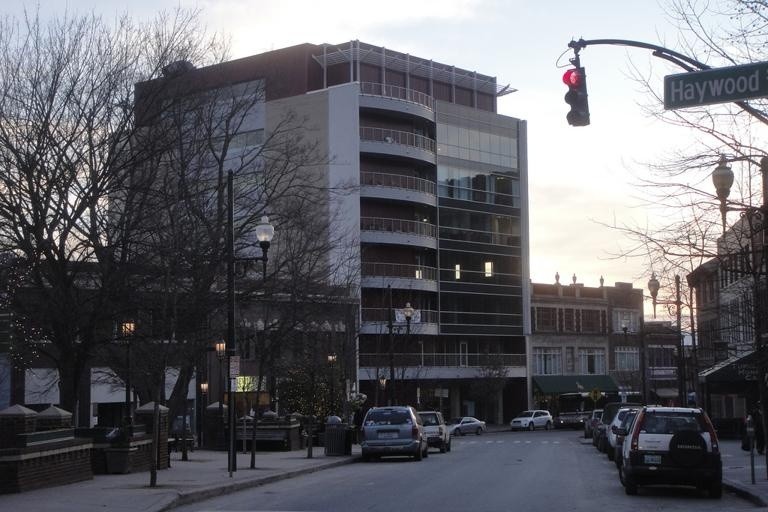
564, 67, 590, 126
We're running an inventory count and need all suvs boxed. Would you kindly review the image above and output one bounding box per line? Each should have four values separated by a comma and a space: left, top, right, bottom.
584, 402, 722, 498
418, 411, 451, 453
360, 404, 428, 461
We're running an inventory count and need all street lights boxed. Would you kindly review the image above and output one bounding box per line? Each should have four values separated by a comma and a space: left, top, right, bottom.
713, 155, 768, 275
123, 316, 135, 435
623, 317, 647, 404
387, 282, 414, 406
227, 168, 274, 473
200, 383, 209, 445
328, 351, 337, 415
648, 272, 683, 408
216, 336, 227, 451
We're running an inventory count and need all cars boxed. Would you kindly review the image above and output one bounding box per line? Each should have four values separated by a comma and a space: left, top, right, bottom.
511, 409, 553, 431
445, 416, 485, 436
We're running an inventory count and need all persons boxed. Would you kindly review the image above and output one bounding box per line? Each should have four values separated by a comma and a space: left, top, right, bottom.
752, 400, 764, 454
745, 410, 755, 450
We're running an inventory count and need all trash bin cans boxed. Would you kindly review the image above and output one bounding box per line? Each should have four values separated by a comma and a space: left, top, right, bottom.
326, 417, 352, 456
